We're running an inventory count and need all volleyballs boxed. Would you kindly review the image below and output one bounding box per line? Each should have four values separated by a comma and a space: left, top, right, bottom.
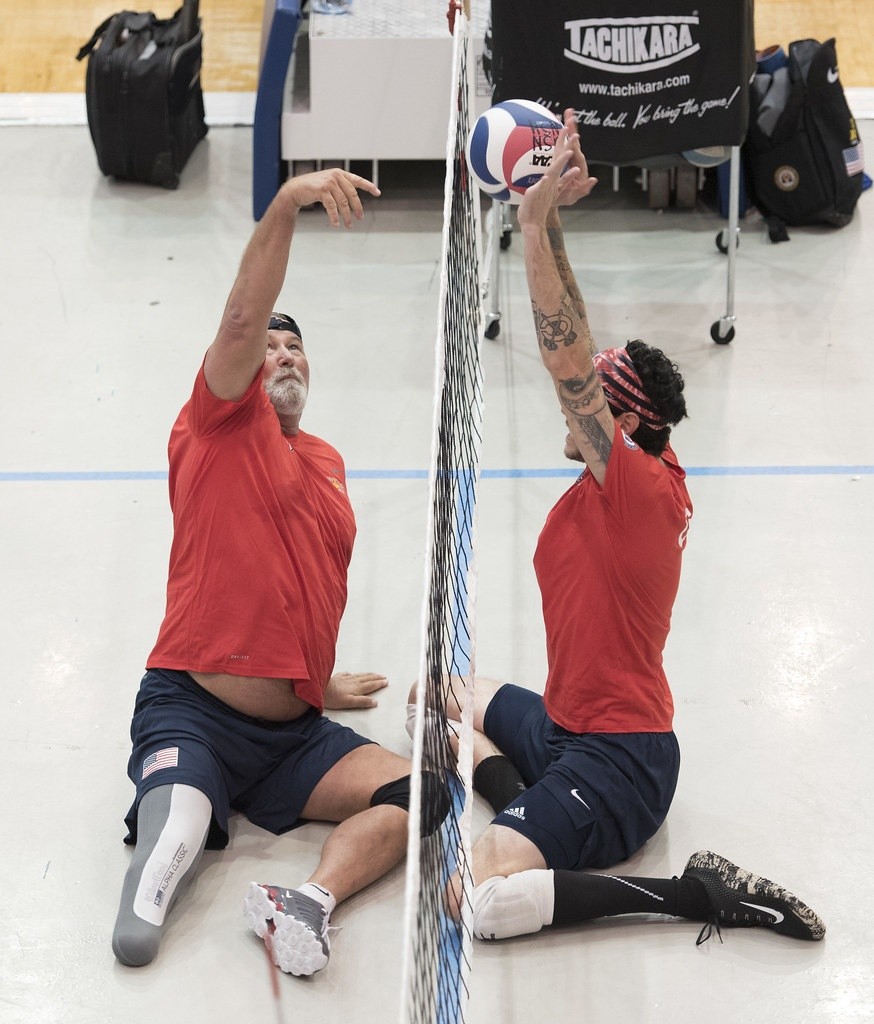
464, 97, 574, 205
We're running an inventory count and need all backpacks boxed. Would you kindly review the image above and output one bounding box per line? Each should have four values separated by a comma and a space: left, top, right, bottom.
744, 36, 873, 243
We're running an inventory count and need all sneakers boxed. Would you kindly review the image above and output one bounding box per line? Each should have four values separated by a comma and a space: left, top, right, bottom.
245, 882, 342, 978
673, 849, 827, 946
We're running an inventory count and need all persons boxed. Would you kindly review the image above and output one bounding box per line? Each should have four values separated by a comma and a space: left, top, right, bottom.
108, 167, 450, 979
453, 107, 832, 945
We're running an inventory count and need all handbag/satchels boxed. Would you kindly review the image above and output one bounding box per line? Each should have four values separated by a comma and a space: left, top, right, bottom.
77, 0, 209, 189
